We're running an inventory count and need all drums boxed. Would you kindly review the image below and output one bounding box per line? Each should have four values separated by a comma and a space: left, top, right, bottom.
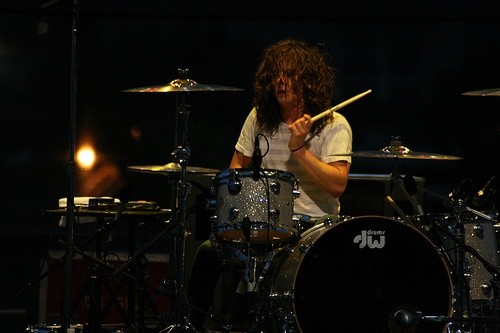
213, 167, 300, 242
445, 211, 500, 299
269, 214, 453, 333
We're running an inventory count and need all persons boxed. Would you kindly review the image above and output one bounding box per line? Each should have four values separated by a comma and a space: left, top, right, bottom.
183, 40, 352, 333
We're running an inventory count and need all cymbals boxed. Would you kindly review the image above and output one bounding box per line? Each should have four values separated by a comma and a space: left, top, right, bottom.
121, 84, 247, 93
127, 163, 223, 177
352, 145, 467, 161
462, 87, 500, 96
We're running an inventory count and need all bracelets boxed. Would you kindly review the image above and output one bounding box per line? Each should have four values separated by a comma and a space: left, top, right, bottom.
290, 143, 305, 152
